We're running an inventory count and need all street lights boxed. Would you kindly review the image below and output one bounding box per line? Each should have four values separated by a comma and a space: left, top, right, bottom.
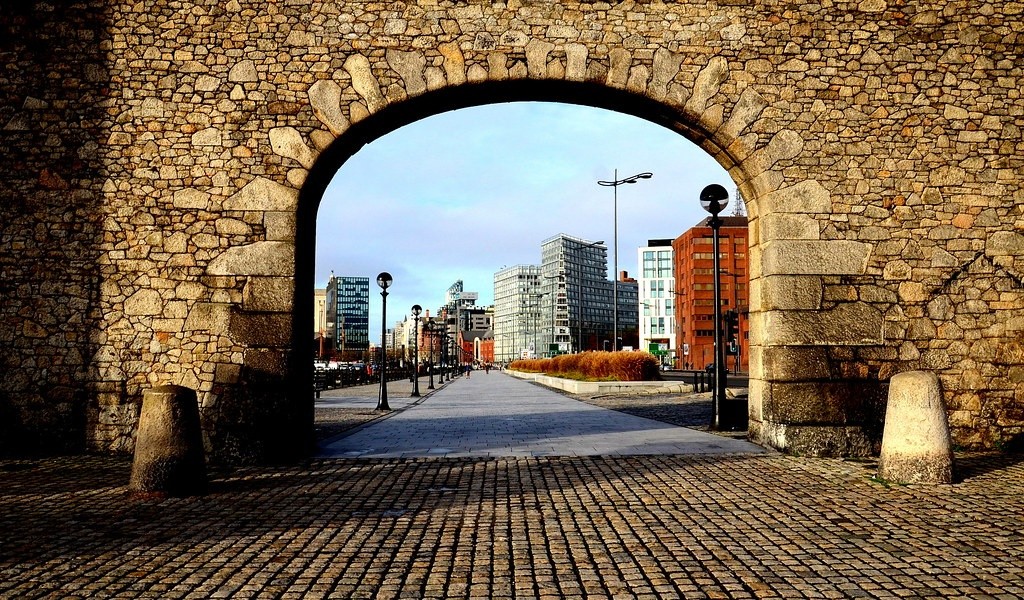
596, 166, 656, 347
410, 301, 478, 401
497, 241, 609, 370
699, 182, 730, 430
376, 271, 394, 411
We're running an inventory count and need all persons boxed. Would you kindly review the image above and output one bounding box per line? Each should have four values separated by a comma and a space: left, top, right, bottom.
366, 363, 371, 382
465, 363, 472, 379
485, 360, 490, 374
